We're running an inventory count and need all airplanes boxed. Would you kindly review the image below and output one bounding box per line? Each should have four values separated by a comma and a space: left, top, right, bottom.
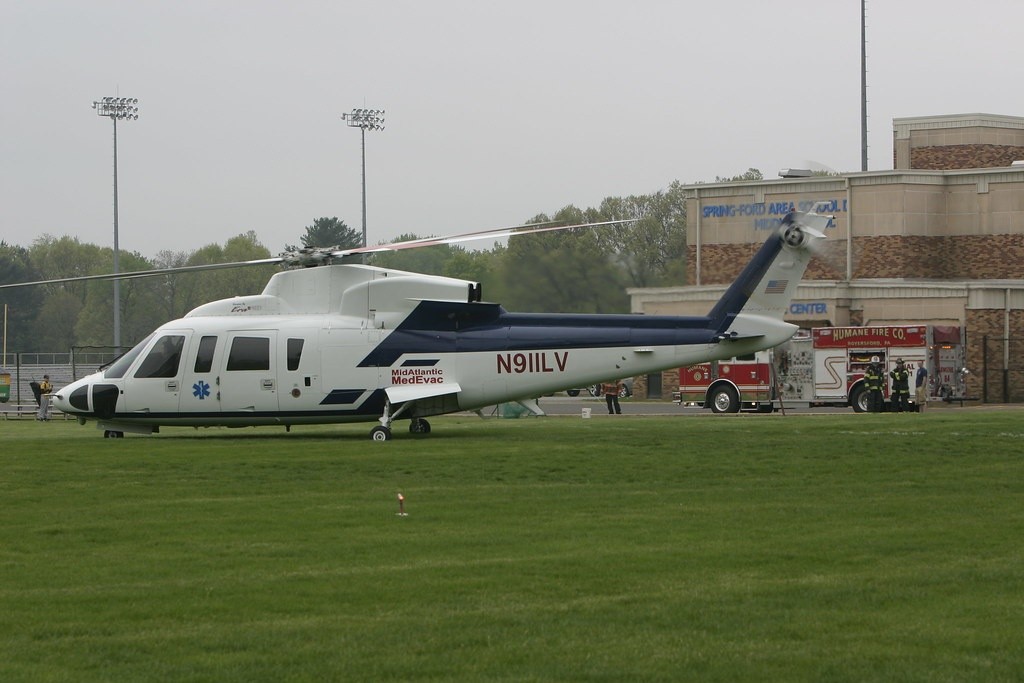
1, 201, 836, 441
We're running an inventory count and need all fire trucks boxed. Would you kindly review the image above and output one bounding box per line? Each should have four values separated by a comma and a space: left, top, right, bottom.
671, 323, 965, 414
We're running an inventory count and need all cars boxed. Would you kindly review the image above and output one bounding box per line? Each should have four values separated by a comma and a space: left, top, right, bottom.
588, 376, 634, 397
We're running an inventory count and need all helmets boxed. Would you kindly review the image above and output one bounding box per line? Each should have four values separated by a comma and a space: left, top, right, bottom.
871, 356, 880, 364
895, 357, 906, 364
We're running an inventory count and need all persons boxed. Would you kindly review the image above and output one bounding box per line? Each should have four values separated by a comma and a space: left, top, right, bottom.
38, 375, 53, 422
890, 358, 910, 413
153, 341, 177, 378
602, 379, 623, 415
915, 360, 928, 414
864, 356, 886, 413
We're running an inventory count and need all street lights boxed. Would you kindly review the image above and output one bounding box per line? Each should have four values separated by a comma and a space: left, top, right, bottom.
91, 96, 140, 369
342, 108, 387, 271
